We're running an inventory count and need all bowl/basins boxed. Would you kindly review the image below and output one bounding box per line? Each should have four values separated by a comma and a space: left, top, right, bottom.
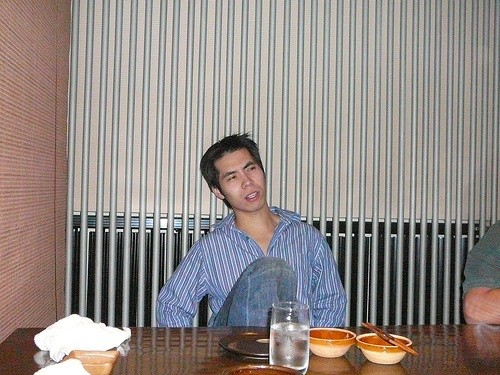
355, 333, 412, 365
221, 363, 302, 375
309, 328, 356, 358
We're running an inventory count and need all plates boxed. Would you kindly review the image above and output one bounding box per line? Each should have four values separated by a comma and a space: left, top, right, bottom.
217, 331, 270, 359
64, 350, 121, 374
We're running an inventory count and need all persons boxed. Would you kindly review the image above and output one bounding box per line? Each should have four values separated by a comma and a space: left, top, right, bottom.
154, 134, 346, 329
462, 220, 500, 325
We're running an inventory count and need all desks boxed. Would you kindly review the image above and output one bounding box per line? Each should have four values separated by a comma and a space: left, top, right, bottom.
0, 321, 500, 375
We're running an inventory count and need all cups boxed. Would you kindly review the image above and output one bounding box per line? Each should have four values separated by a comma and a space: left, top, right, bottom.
269, 301, 311, 375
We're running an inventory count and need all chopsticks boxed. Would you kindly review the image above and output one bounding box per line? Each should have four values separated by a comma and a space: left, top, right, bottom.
361, 322, 419, 356
